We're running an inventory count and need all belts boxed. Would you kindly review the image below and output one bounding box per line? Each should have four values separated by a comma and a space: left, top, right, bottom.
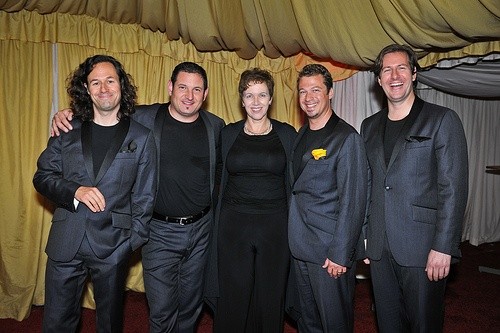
152, 206, 210, 226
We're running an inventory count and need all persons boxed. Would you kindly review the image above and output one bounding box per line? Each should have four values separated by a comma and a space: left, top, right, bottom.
51, 62, 227, 333
354, 43, 469, 333
288, 64, 368, 333
33, 55, 158, 333
203, 67, 298, 333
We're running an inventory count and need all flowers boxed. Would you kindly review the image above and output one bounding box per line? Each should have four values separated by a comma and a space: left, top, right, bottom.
312, 149, 327, 161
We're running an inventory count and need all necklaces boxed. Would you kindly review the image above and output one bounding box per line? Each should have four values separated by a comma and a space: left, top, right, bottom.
244, 122, 271, 134
